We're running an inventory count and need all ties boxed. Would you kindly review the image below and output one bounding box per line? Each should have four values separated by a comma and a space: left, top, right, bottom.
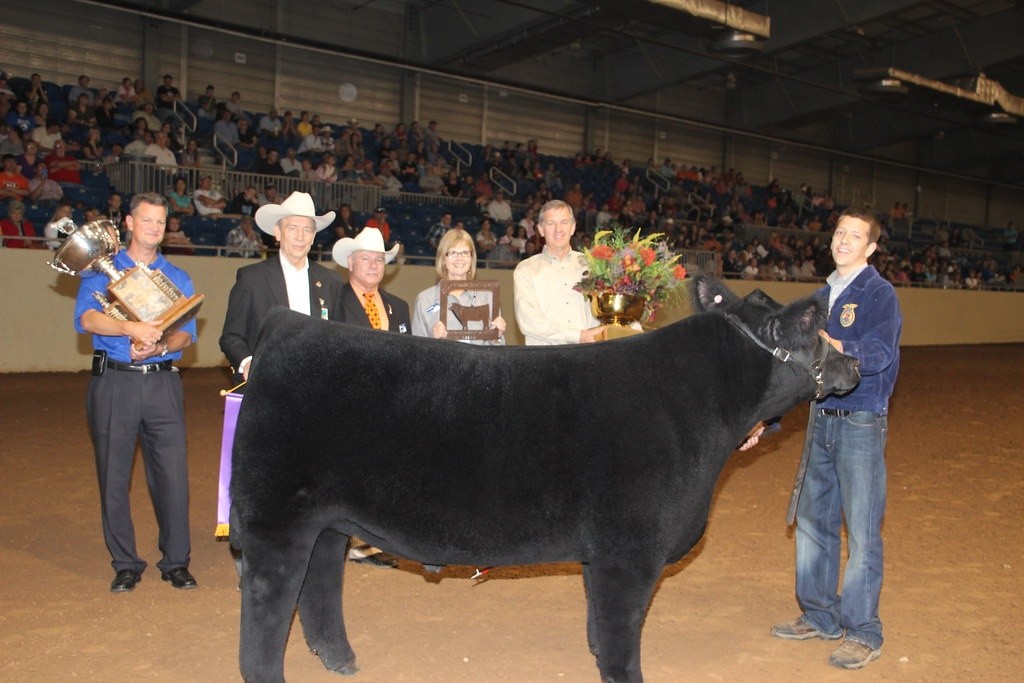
364, 293, 382, 329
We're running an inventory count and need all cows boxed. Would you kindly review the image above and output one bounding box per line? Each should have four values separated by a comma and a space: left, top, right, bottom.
229, 273, 865, 683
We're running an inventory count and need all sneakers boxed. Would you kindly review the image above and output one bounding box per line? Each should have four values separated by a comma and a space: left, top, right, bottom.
829, 640, 881, 669
772, 617, 843, 641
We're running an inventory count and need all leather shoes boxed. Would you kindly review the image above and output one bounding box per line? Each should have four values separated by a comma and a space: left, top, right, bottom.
111, 569, 141, 592
161, 567, 197, 589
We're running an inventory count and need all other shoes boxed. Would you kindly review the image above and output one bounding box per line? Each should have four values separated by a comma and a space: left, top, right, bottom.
349, 544, 399, 567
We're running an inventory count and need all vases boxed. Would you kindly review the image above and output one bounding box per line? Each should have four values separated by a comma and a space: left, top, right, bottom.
590, 285, 646, 325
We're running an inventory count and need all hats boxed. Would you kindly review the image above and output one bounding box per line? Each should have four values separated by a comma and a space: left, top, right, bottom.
255, 191, 336, 235
332, 227, 400, 268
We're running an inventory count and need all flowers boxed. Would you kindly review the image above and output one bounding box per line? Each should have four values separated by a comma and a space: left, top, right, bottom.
571, 222, 691, 323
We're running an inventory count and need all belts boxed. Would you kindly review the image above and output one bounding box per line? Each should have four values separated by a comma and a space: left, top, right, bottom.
108, 359, 172, 374
820, 408, 852, 417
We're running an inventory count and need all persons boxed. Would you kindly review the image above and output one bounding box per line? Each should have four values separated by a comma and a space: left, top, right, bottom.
512, 199, 642, 346
331, 225, 413, 568
218, 188, 336, 587
76, 191, 199, 593
413, 228, 507, 348
738, 206, 903, 670
1, 71, 1024, 295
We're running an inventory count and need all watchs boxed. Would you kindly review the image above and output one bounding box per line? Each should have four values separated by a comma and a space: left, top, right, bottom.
159, 342, 168, 358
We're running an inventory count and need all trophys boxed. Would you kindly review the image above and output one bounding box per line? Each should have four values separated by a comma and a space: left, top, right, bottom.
572, 230, 686, 339
45, 217, 204, 349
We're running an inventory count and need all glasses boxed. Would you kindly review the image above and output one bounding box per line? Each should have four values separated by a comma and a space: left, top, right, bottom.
445, 251, 472, 257
